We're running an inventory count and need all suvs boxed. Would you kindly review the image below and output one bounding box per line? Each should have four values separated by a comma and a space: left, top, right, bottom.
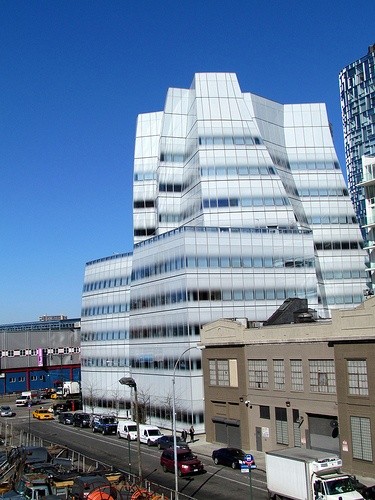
160, 448, 204, 477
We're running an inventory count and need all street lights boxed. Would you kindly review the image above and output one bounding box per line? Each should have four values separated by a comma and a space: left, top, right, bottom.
172, 345, 206, 500
119, 376, 143, 485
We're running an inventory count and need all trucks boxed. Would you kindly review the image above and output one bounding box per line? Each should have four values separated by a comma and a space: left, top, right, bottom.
15, 390, 41, 407
264, 447, 366, 500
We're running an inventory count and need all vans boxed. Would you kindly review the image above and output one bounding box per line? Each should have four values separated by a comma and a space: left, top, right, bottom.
138, 425, 165, 447
72, 413, 91, 428
117, 421, 139, 441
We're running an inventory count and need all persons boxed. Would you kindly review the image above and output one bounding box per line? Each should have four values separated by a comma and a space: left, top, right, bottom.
190, 426, 195, 444
182, 428, 187, 442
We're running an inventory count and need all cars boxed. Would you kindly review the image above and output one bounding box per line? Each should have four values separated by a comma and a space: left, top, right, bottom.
212, 447, 255, 469
89, 414, 118, 435
0, 405, 13, 417
155, 435, 188, 451
39, 387, 63, 399
32, 409, 53, 420
0, 445, 171, 500
58, 413, 74, 425
